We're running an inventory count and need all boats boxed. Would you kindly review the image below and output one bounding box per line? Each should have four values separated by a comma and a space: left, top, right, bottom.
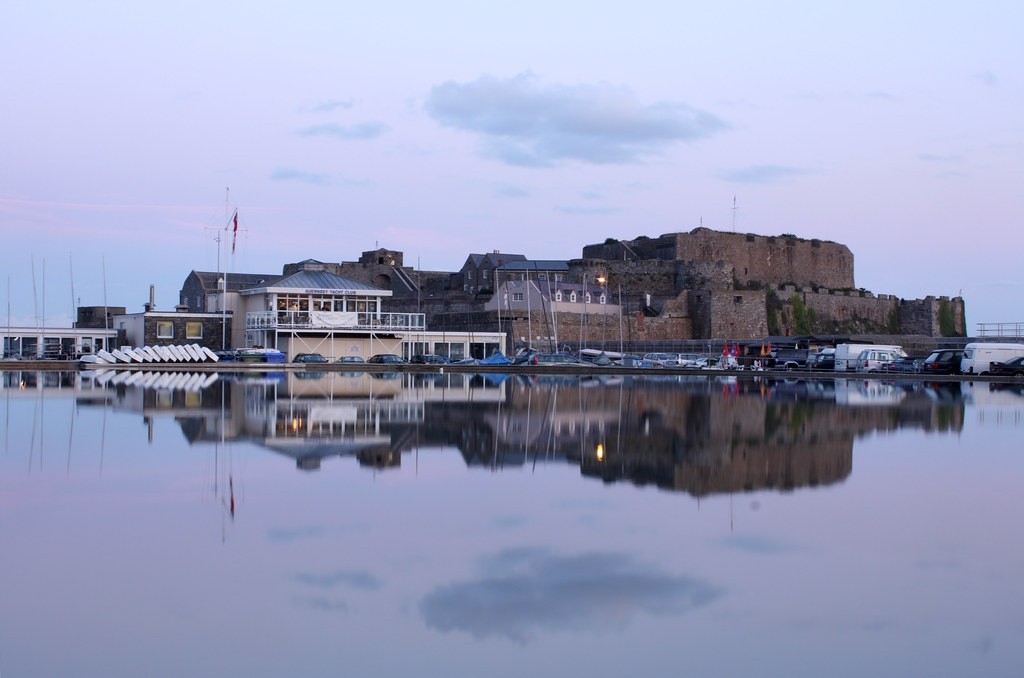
700, 354, 763, 371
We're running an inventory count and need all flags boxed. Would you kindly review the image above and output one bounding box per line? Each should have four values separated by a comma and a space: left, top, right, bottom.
233, 213, 238, 252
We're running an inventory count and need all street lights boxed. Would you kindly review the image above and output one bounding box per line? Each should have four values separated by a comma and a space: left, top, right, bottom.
596, 274, 607, 353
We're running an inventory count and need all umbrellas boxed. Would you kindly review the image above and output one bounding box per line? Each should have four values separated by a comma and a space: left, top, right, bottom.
723, 340, 740, 357
762, 341, 771, 358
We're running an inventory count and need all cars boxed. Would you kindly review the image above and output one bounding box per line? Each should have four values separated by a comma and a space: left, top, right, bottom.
922, 349, 964, 375
292, 353, 329, 363
990, 356, 1024, 376
368, 354, 404, 363
336, 356, 364, 364
889, 357, 926, 375
806, 353, 835, 368
410, 354, 447, 365
769, 350, 808, 371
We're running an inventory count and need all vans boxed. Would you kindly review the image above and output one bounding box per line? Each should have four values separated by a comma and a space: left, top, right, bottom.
855, 349, 902, 373
643, 352, 666, 367
530, 354, 598, 367
960, 342, 1024, 376
834, 343, 909, 371
666, 353, 708, 366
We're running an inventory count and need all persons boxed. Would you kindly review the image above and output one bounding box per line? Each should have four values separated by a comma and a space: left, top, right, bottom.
754, 359, 760, 368
